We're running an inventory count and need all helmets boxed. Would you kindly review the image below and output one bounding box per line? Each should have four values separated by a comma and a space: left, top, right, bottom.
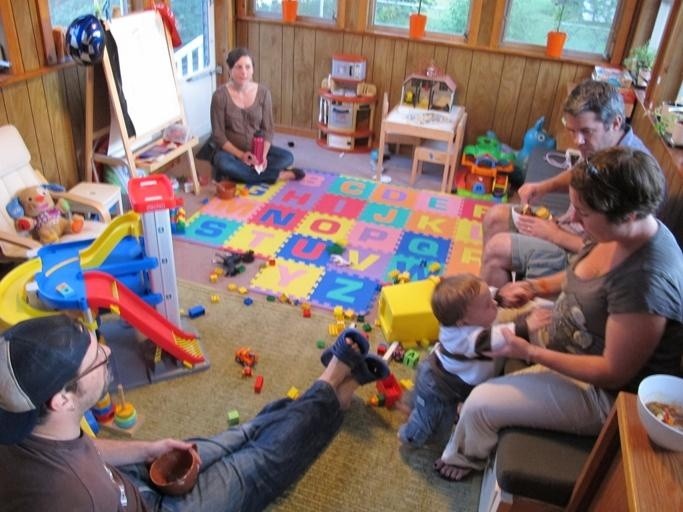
65, 15, 105, 67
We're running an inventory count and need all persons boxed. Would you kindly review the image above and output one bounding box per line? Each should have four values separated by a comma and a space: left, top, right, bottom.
432, 146, 682, 481
478, 81, 669, 286
209, 48, 305, 182
395, 271, 554, 445
1, 314, 392, 510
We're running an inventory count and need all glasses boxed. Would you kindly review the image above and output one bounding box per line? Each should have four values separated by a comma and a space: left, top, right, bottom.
64, 342, 107, 388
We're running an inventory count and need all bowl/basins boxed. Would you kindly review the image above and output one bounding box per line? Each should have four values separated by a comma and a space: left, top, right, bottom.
511, 204, 553, 238
636, 373, 683, 452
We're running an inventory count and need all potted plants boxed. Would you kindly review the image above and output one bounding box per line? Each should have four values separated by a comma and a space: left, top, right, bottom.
409, 0, 429, 38
621, 39, 655, 87
641, 99, 683, 147
545, 0, 581, 59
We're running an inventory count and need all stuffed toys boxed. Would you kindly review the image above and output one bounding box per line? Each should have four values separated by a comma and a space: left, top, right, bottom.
4, 181, 82, 244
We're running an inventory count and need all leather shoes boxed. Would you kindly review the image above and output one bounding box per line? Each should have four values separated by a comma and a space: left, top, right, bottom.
285, 169, 305, 181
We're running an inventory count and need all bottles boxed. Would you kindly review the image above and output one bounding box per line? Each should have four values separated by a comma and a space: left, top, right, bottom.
252, 130, 265, 165
426, 59, 439, 79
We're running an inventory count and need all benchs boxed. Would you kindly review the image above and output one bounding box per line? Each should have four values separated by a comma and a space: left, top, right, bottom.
478, 147, 599, 512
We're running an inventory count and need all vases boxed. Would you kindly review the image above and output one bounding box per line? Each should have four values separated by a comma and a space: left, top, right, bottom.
282, 0, 298, 23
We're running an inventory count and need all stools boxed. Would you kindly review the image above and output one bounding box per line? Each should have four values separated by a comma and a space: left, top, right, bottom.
65, 181, 123, 221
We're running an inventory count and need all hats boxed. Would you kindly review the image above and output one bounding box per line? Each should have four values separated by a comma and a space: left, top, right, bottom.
0, 314, 90, 445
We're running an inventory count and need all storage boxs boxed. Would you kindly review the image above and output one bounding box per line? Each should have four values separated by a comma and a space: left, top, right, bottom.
332, 54, 367, 81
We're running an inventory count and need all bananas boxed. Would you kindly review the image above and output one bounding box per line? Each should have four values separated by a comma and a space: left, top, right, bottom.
521, 203, 549, 220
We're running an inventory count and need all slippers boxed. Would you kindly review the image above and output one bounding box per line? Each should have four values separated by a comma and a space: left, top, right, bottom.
321, 329, 369, 371
353, 354, 389, 385
433, 459, 474, 481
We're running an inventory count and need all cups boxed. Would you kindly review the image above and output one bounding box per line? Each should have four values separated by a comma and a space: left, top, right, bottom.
149, 442, 201, 496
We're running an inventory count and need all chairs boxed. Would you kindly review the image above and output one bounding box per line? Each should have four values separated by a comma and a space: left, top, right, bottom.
410, 112, 468, 195
0, 124, 112, 269
381, 92, 423, 156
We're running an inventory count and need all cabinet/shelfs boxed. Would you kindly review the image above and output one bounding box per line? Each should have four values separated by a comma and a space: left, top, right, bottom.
315, 77, 378, 153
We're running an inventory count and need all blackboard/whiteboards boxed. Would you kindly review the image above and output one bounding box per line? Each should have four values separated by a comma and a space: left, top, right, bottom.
102, 10, 185, 143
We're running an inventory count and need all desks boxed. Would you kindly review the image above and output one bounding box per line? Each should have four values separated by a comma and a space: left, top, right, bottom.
559, 391, 683, 512
375, 101, 466, 194
